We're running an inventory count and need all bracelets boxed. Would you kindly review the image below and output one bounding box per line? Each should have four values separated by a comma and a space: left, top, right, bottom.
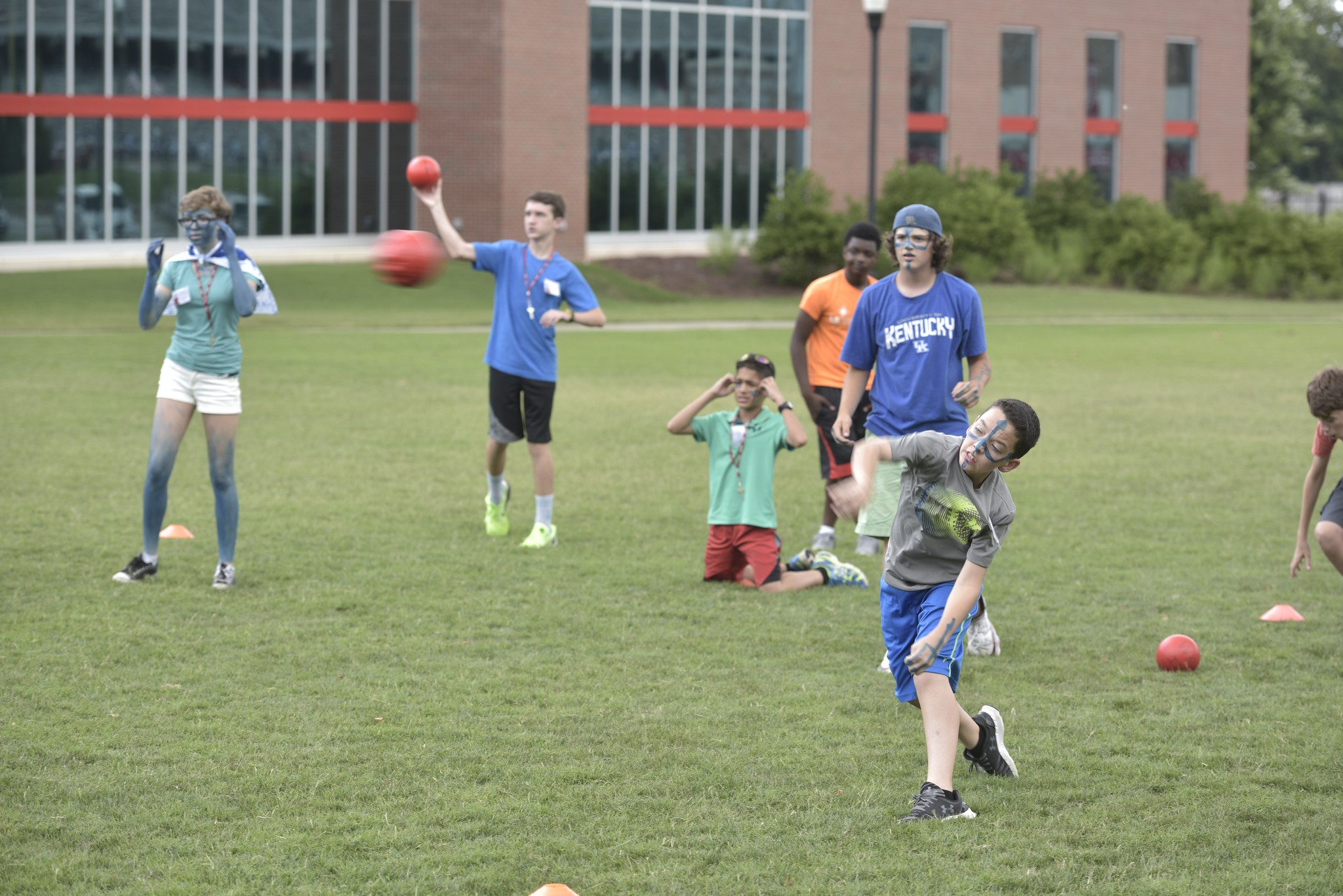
560, 301, 575, 322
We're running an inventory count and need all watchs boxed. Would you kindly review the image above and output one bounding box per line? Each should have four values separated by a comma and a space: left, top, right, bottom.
779, 401, 793, 413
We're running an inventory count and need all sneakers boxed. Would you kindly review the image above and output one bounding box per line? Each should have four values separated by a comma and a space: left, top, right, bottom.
483, 479, 510, 537
962, 705, 1020, 779
788, 547, 815, 570
812, 530, 836, 551
518, 521, 558, 550
966, 592, 1001, 657
112, 553, 158, 583
212, 561, 237, 590
895, 782, 977, 833
854, 535, 880, 557
810, 550, 869, 589
876, 649, 892, 673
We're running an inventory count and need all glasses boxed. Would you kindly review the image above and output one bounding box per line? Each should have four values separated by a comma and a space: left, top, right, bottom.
177, 217, 220, 229
739, 354, 774, 374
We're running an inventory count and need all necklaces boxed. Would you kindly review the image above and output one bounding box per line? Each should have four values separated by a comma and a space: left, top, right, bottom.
192, 262, 220, 349
728, 414, 751, 501
522, 241, 558, 321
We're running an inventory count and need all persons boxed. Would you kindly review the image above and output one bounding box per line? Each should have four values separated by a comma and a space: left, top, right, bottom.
1289, 366, 1343, 581
830, 204, 1002, 675
412, 176, 606, 550
666, 352, 870, 593
111, 185, 278, 591
851, 399, 1042, 827
789, 222, 882, 558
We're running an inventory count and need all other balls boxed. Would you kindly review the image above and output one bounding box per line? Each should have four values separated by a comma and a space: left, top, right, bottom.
1156, 633, 1201, 674
405, 156, 442, 189
376, 230, 447, 291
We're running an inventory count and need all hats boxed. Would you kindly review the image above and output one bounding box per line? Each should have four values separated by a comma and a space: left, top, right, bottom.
892, 204, 943, 237
